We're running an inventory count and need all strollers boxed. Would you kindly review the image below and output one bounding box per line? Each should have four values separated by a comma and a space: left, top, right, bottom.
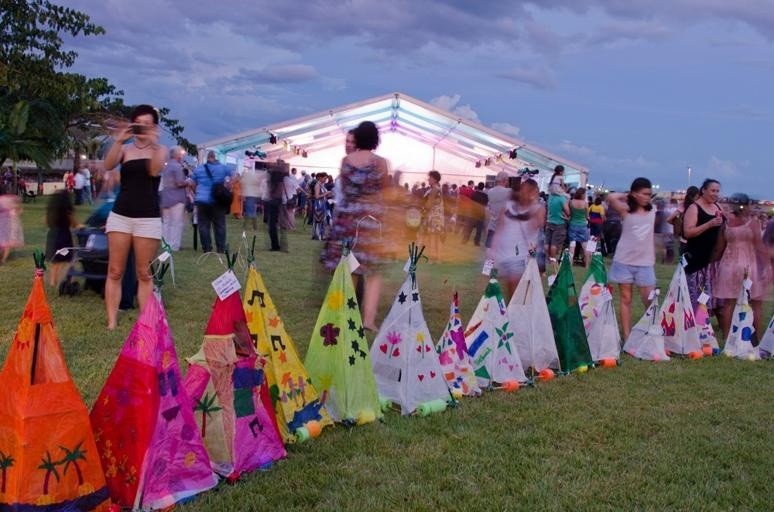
57, 197, 142, 313
597, 211, 623, 256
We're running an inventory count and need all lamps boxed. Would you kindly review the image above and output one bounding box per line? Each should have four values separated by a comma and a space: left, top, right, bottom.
266, 131, 309, 161
474, 147, 518, 170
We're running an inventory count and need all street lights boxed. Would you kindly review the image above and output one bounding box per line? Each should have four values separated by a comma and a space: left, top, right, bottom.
652, 183, 659, 191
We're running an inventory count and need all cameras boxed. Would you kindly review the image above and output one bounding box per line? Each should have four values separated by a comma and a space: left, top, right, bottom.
132, 125, 146, 134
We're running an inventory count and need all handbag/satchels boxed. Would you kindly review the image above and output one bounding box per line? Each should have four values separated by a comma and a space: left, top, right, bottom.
210, 183, 233, 206
314, 208, 323, 223
287, 199, 295, 210
